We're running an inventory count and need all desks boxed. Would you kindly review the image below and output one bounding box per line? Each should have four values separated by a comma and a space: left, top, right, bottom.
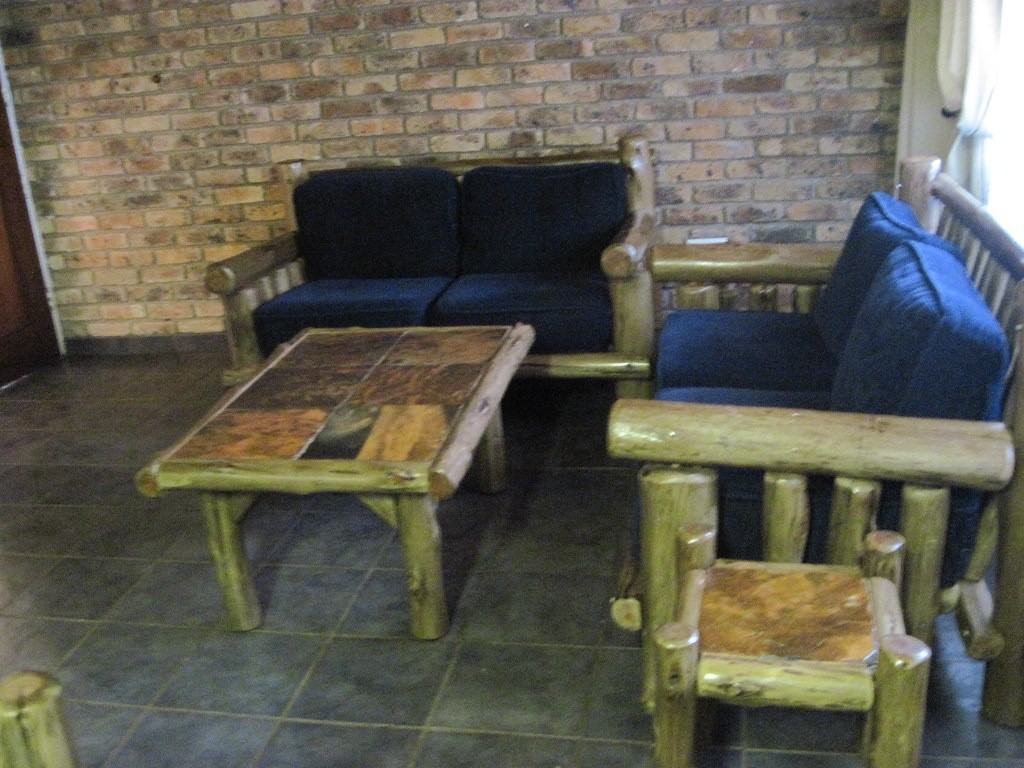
132, 317, 536, 643
646, 519, 934, 768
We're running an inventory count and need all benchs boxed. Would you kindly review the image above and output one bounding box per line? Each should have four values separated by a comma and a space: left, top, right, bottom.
204, 134, 663, 447
605, 177, 1024, 732
